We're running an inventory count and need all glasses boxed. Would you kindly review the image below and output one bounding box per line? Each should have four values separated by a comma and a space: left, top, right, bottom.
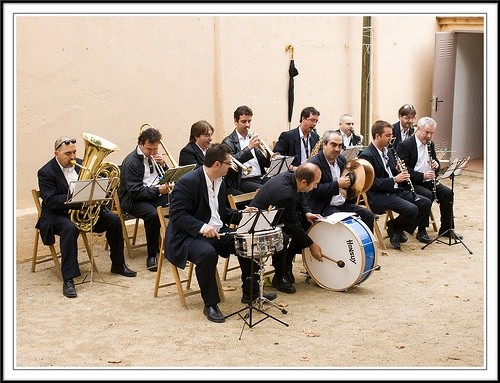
55, 138, 76, 150
305, 118, 320, 124
219, 161, 232, 166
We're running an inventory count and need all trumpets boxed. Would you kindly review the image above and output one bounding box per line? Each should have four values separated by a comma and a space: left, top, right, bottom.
245, 126, 277, 163
209, 141, 253, 178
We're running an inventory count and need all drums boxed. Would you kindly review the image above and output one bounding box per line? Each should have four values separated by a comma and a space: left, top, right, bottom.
234, 226, 286, 257
301, 213, 377, 295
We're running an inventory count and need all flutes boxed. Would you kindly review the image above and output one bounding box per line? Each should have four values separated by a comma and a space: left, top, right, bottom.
387, 140, 420, 202
426, 138, 439, 202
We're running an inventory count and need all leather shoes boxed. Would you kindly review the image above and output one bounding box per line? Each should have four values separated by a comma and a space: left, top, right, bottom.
240, 292, 276, 303
284, 263, 294, 283
111, 262, 137, 277
399, 231, 408, 242
203, 304, 225, 323
416, 230, 432, 244
146, 255, 157, 270
272, 273, 296, 294
438, 228, 463, 240
387, 219, 401, 250
62, 280, 77, 298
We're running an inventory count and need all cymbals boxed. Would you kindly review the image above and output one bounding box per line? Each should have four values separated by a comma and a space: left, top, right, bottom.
353, 157, 375, 196
337, 161, 364, 200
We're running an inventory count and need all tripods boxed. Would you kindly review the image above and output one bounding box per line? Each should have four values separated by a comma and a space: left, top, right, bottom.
244, 254, 287, 319
421, 157, 473, 255
64, 178, 130, 291
224, 209, 289, 339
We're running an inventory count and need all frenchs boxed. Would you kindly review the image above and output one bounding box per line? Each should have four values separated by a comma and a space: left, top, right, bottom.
66, 132, 120, 232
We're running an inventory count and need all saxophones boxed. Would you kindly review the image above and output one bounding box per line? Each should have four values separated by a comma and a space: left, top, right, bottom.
305, 125, 325, 161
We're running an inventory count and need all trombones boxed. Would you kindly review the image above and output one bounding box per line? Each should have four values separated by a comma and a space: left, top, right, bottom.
138, 122, 179, 195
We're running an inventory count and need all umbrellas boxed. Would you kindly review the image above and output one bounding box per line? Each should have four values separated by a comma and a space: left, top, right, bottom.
286, 44, 299, 130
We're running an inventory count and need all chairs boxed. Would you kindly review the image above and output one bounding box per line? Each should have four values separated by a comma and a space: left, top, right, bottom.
357, 190, 394, 250
223, 187, 276, 283
104, 190, 161, 259
154, 206, 226, 308
30, 189, 100, 280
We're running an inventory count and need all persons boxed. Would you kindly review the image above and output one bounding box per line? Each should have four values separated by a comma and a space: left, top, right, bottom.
273, 107, 320, 166
338, 114, 361, 148
178, 121, 240, 209
391, 104, 416, 146
304, 131, 381, 271
118, 128, 171, 271
221, 105, 272, 192
248, 163, 323, 293
396, 116, 463, 243
166, 143, 277, 323
359, 120, 430, 249
35, 136, 137, 298
